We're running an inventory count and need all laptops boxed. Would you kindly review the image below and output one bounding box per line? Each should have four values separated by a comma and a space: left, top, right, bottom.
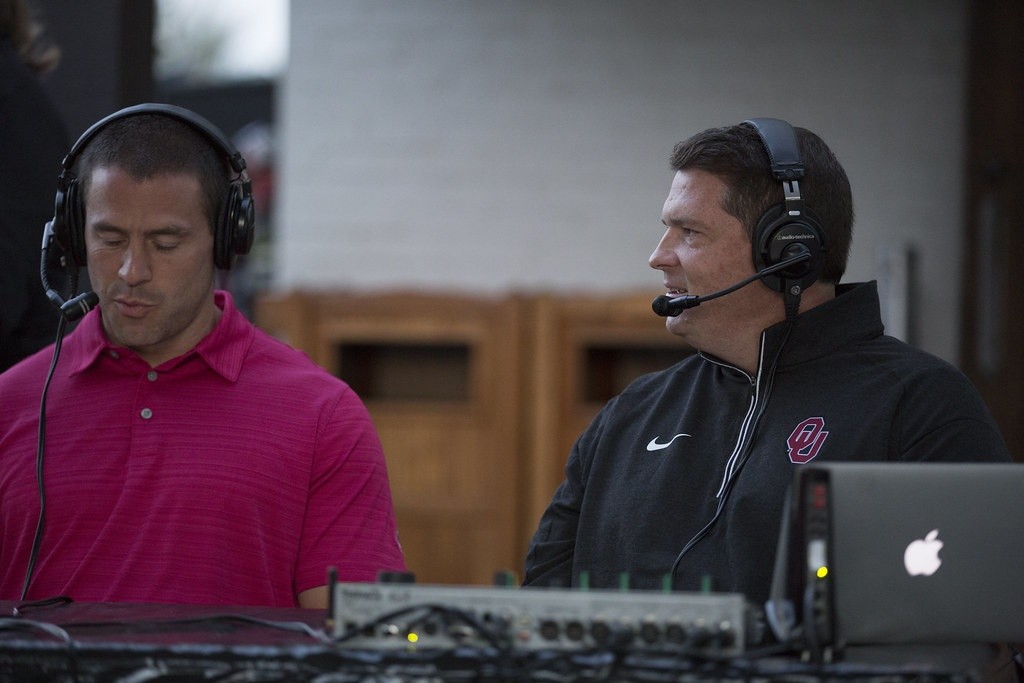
770, 461, 1024, 649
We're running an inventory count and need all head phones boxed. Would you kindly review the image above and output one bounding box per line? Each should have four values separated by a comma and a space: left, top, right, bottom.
739, 118, 827, 294
52, 104, 255, 271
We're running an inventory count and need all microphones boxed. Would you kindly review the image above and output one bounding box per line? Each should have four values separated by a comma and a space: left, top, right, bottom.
41, 223, 100, 321
652, 250, 812, 317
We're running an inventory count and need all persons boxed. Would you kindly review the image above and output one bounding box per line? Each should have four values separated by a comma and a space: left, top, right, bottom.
0, 103, 407, 611
518, 117, 1013, 611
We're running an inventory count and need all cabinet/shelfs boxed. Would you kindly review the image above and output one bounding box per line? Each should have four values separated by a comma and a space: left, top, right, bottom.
231, 287, 693, 587
